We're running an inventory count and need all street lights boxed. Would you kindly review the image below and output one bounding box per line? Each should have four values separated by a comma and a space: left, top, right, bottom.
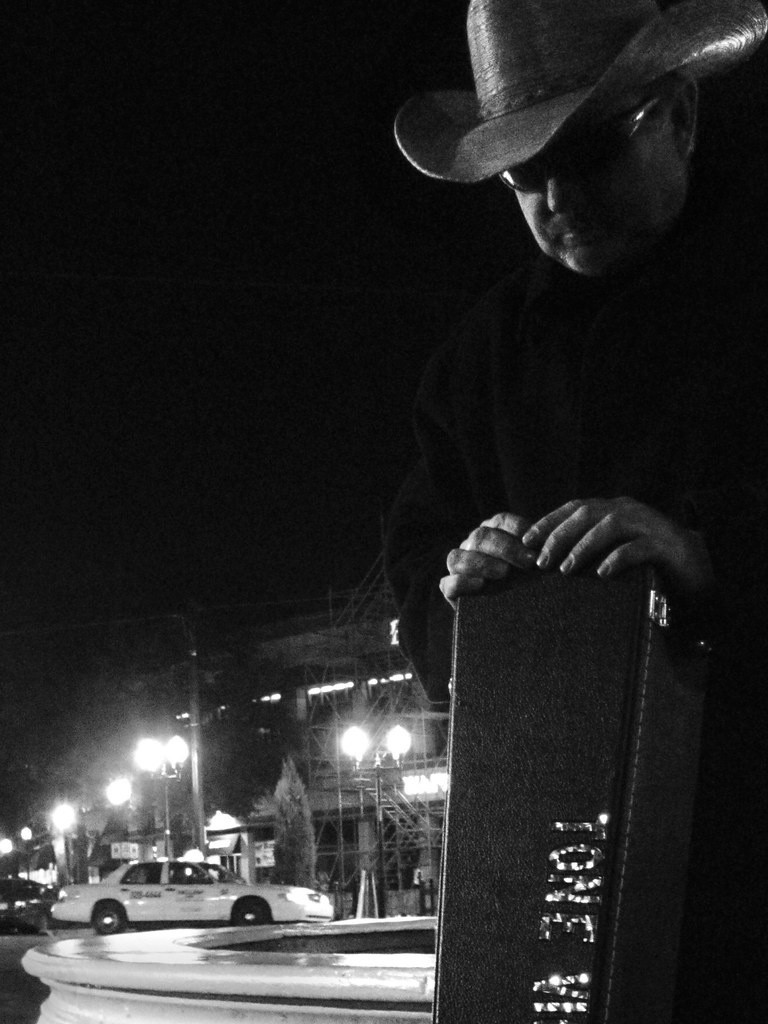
132, 733, 190, 857
51, 803, 75, 885
19, 827, 33, 881
104, 776, 133, 866
338, 725, 413, 918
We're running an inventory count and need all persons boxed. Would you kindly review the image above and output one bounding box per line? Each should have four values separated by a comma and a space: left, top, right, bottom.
383, 0, 768, 1024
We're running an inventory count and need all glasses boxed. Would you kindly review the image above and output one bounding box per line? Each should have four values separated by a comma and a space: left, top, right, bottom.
498, 94, 666, 193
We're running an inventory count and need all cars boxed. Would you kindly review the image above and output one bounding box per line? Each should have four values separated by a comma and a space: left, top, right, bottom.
50, 857, 337, 936
0, 875, 60, 936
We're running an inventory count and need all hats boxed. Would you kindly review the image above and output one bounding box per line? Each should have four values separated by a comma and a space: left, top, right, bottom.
393, 0, 768, 181
